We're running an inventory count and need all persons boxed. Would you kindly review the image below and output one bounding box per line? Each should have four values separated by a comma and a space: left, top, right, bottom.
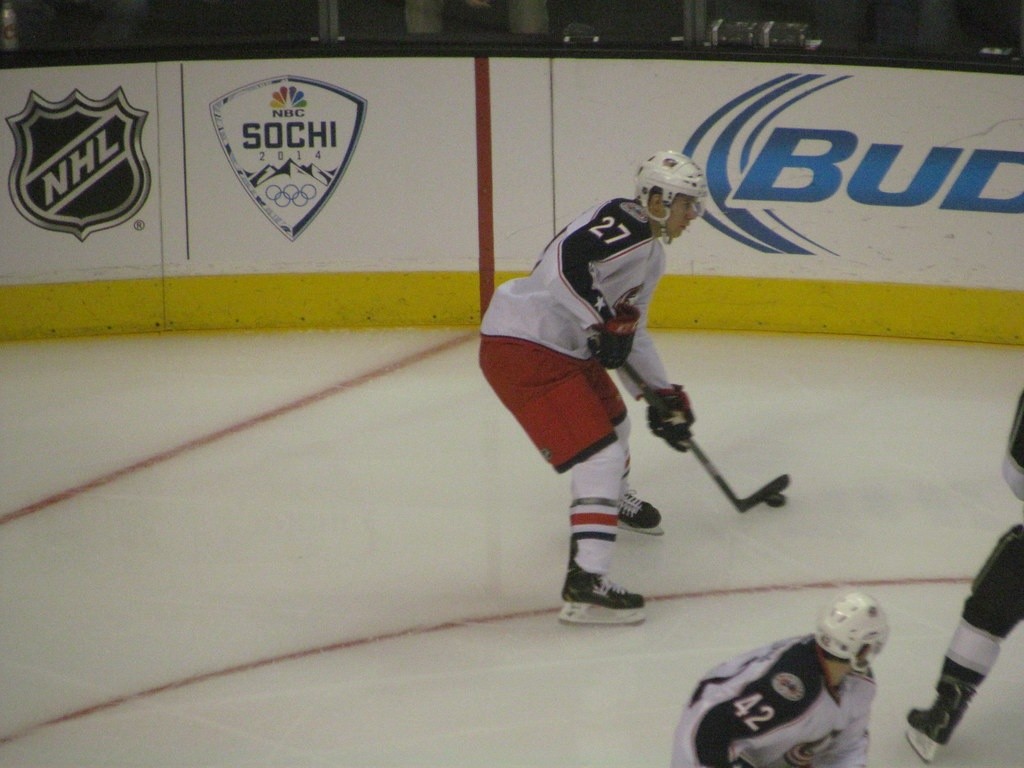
477, 149, 710, 625
671, 592, 890, 768
905, 393, 1024, 763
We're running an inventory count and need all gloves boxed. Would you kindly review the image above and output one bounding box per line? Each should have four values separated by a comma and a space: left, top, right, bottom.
647, 383, 695, 452
588, 305, 640, 368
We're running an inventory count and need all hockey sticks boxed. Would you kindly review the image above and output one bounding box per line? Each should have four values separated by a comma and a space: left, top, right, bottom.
624, 361, 791, 514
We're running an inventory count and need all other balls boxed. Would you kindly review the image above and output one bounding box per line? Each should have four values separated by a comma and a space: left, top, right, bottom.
765, 492, 786, 507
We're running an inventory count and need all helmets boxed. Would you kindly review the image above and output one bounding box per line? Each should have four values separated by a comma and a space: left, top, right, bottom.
817, 591, 887, 669
637, 151, 706, 211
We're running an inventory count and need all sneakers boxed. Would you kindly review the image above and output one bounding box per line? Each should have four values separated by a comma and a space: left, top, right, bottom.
617, 487, 665, 536
905, 680, 977, 763
559, 559, 647, 626
